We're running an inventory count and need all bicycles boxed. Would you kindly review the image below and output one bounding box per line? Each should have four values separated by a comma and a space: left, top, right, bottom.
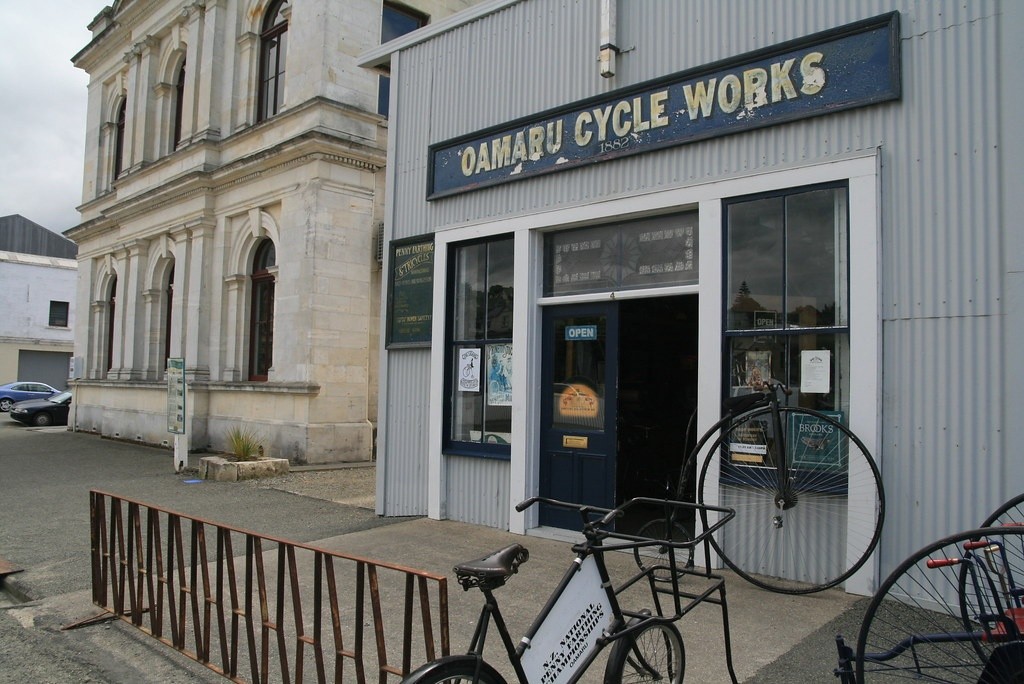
395, 495, 743, 684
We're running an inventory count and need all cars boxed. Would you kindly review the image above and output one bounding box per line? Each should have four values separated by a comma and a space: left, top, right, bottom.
10, 390, 72, 426
0, 382, 63, 412
